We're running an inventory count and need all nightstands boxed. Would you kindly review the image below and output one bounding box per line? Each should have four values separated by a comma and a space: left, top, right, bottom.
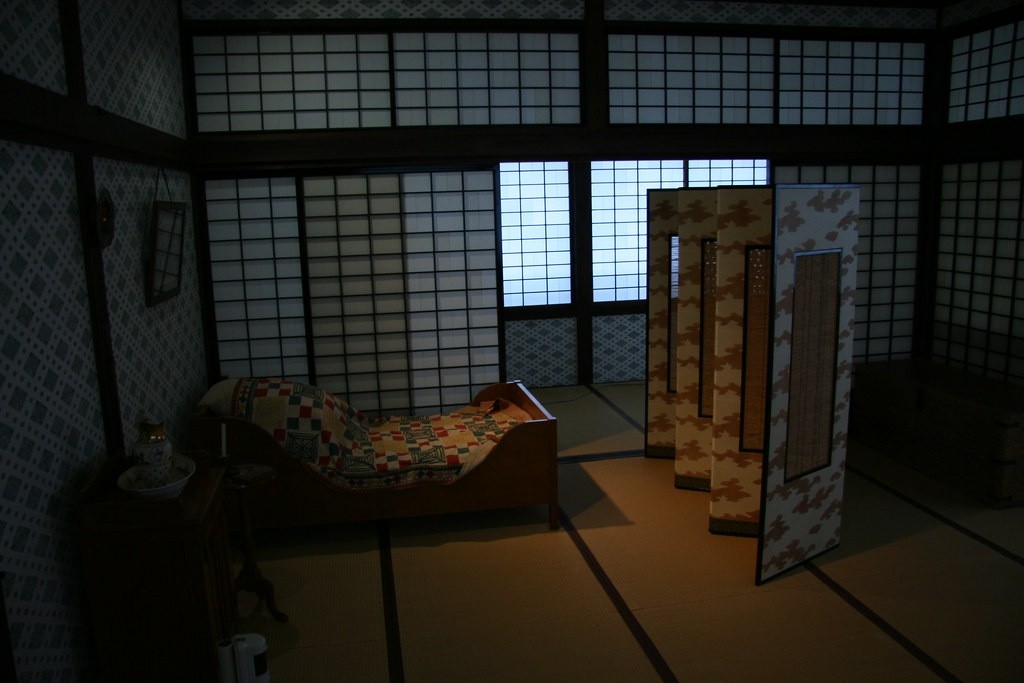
67, 464, 238, 683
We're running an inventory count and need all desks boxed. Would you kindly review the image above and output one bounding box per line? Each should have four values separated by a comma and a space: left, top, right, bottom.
852, 357, 1024, 510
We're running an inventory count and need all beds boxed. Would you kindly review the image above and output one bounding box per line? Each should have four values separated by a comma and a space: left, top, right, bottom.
194, 374, 562, 531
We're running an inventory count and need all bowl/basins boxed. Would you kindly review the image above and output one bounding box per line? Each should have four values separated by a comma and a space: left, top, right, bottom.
116, 455, 196, 503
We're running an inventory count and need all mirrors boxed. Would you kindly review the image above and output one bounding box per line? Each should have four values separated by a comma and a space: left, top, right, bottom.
148, 199, 189, 302
